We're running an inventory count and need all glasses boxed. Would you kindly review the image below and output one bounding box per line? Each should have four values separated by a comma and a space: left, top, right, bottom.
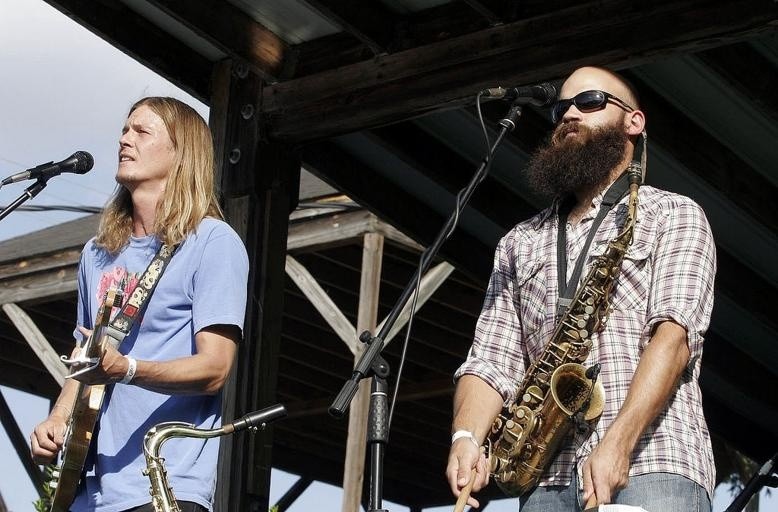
549, 89, 634, 124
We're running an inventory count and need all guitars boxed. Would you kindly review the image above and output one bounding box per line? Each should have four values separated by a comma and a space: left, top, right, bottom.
50, 292, 124, 511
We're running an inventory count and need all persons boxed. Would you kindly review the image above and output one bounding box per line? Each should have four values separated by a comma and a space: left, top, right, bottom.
445, 67, 720, 512
29, 97, 250, 512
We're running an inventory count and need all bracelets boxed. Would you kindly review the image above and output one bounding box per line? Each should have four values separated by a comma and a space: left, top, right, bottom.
451, 430, 478, 447
119, 359, 136, 384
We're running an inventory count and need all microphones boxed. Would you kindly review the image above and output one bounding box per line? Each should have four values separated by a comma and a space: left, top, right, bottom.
3, 149, 97, 179
482, 81, 558, 106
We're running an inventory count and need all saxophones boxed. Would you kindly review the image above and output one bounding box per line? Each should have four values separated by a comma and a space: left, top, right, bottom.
484, 162, 642, 498
